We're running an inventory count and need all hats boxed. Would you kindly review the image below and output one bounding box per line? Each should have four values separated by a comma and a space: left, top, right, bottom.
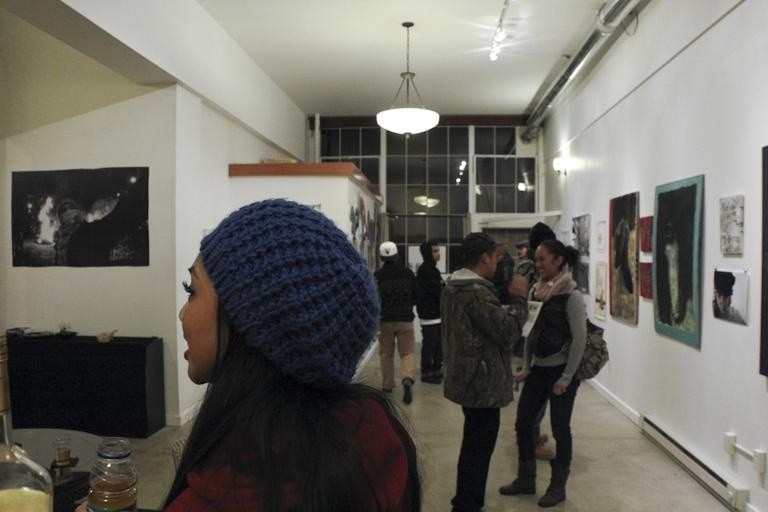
201, 198, 381, 385
459, 232, 507, 261
378, 242, 398, 261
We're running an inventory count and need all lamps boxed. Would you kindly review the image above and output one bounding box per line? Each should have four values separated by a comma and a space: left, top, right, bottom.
414, 183, 440, 211
549, 155, 564, 177
515, 175, 535, 196
487, 2, 513, 62
518, 0, 648, 142
455, 158, 470, 186
373, 21, 443, 141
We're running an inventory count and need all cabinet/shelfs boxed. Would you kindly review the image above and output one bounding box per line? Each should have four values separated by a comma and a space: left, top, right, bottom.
3, 326, 167, 440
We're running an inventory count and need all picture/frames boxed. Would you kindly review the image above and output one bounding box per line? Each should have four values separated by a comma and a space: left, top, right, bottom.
650, 174, 706, 352
607, 191, 643, 326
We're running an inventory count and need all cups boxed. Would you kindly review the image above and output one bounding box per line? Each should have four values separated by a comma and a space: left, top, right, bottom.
52, 436, 75, 468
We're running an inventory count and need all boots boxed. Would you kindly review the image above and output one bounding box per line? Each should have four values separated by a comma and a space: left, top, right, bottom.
538, 460, 571, 507
498, 458, 537, 496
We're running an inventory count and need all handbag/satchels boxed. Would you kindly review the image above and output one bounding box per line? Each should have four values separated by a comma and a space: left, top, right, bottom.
559, 322, 608, 381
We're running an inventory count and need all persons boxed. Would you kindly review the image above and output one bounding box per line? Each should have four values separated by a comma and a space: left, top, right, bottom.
497, 236, 588, 507
414, 237, 449, 386
509, 222, 559, 460
438, 231, 530, 511
713, 271, 746, 326
492, 237, 516, 300
510, 240, 538, 290
159, 196, 425, 510
371, 238, 419, 405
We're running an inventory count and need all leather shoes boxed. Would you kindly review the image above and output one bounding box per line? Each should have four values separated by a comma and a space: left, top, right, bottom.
400, 378, 412, 405
419, 375, 443, 385
535, 445, 555, 460
537, 434, 547, 445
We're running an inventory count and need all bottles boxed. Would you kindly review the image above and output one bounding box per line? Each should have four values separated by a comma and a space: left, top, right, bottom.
87, 434, 137, 509
0, 335, 54, 512
